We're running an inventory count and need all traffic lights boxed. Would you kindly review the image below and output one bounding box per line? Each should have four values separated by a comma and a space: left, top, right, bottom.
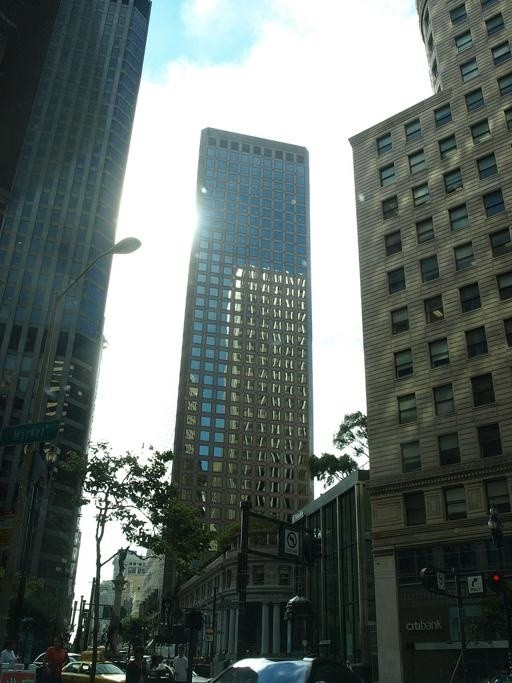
492, 574, 506, 593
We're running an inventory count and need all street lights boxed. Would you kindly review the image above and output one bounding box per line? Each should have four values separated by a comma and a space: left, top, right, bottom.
1, 237, 142, 627
487, 505, 512, 653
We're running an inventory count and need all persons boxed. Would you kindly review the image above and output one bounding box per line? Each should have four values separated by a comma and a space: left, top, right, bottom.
126, 646, 149, 683
173, 644, 188, 683
0, 640, 20, 669
43, 637, 69, 683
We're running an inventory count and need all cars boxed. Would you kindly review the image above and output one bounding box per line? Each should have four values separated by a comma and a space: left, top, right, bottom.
128, 654, 213, 683
206, 657, 362, 683
61, 650, 127, 683
33, 653, 82, 674
